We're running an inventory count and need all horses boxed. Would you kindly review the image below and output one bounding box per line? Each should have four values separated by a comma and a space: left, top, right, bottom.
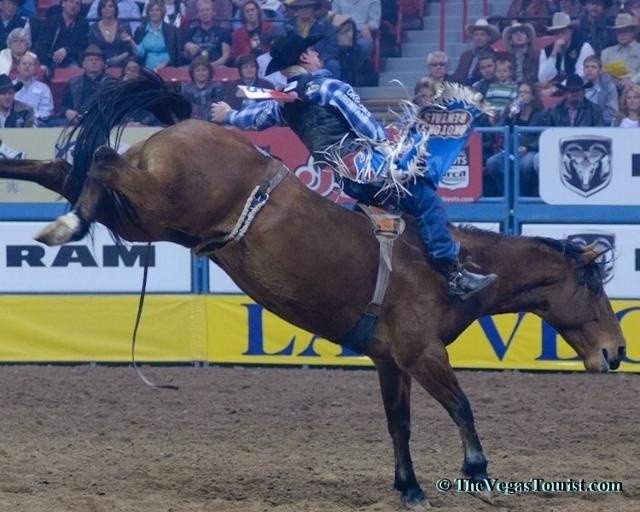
0, 64, 626, 511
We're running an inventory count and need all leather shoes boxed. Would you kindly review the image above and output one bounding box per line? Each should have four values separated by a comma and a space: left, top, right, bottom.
446, 269, 499, 303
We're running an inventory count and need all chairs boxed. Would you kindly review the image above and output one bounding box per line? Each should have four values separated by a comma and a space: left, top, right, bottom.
458, 27, 591, 127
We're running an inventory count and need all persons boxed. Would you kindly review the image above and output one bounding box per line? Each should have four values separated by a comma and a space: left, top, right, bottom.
1, 1, 640, 199
210, 30, 498, 303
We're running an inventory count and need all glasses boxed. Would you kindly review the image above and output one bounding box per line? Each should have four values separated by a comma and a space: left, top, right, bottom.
518, 88, 533, 94
427, 62, 446, 67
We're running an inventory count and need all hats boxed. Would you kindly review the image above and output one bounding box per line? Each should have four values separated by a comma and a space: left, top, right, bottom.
545, 10, 582, 34
288, 0, 320, 8
264, 34, 324, 77
553, 73, 593, 92
0, 73, 24, 94
464, 17, 537, 47
606, 12, 640, 33
78, 43, 107, 66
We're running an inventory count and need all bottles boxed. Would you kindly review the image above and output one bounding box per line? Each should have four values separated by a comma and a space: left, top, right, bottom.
508, 97, 525, 118
